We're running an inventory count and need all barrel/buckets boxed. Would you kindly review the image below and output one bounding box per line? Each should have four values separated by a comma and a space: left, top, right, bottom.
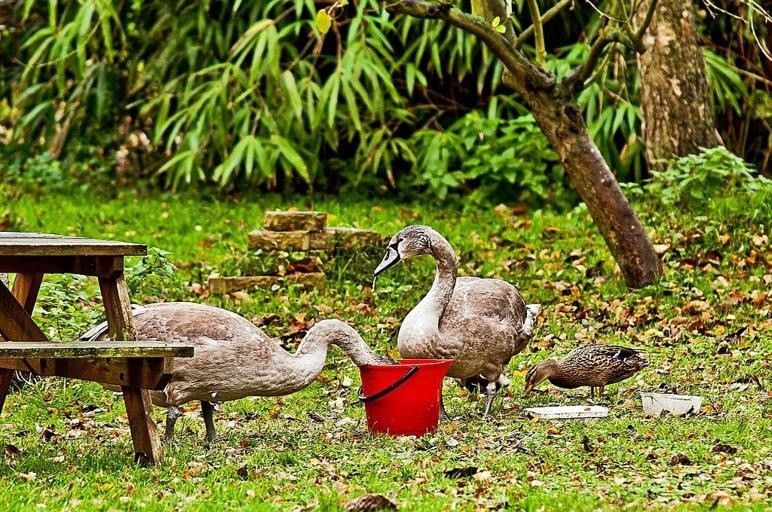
360, 358, 455, 434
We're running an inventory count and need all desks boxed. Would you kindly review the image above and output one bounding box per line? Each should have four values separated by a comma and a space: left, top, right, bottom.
0, 232, 164, 469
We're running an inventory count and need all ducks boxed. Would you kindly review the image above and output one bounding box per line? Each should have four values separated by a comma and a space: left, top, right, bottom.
374, 224, 542, 423
74, 302, 400, 442
520, 344, 650, 398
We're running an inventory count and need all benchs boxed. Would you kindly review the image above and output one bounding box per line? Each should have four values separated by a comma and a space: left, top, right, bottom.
0, 340, 196, 475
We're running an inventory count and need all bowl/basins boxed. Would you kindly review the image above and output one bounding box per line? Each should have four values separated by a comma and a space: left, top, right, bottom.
639, 392, 702, 416
